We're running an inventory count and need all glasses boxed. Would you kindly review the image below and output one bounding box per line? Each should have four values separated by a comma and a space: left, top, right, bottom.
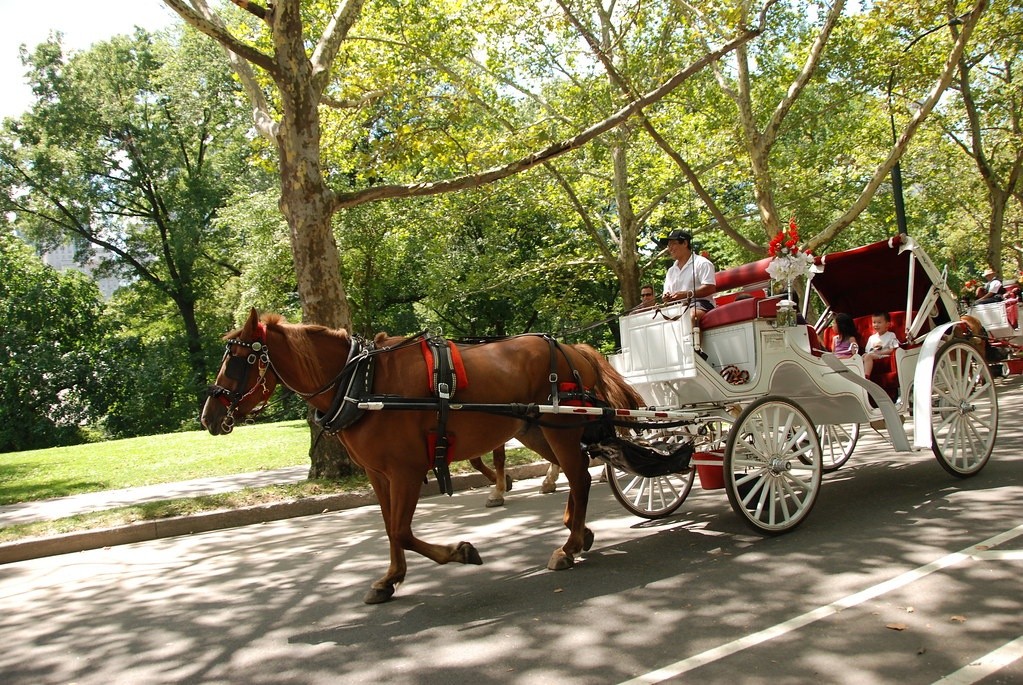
641, 293, 653, 297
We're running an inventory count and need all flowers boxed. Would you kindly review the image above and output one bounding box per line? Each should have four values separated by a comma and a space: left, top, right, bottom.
961, 276, 984, 299
763, 214, 816, 284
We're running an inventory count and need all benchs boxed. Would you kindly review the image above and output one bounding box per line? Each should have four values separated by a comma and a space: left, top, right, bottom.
976, 284, 1021, 329
695, 254, 795, 331
797, 307, 931, 389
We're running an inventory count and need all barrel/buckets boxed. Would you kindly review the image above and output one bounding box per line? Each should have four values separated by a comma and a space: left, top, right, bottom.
1007, 358, 1023, 375
691, 449, 726, 491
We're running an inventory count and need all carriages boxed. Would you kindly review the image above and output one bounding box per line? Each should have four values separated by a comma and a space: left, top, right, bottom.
948, 277, 1023, 388
198, 232, 1023, 604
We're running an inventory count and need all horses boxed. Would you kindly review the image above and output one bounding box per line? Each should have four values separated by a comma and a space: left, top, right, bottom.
200, 306, 647, 604
948, 315, 988, 397
374, 332, 633, 508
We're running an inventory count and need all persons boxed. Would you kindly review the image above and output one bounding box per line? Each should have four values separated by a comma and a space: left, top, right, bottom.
733, 288, 829, 357
629, 286, 655, 315
863, 309, 900, 379
661, 229, 716, 362
975, 268, 1004, 305
831, 312, 860, 359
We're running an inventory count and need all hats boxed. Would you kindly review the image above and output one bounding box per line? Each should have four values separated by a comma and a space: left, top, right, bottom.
660, 229, 691, 245
981, 268, 996, 277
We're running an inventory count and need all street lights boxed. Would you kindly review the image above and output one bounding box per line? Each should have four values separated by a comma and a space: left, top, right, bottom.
887, 8, 973, 236
